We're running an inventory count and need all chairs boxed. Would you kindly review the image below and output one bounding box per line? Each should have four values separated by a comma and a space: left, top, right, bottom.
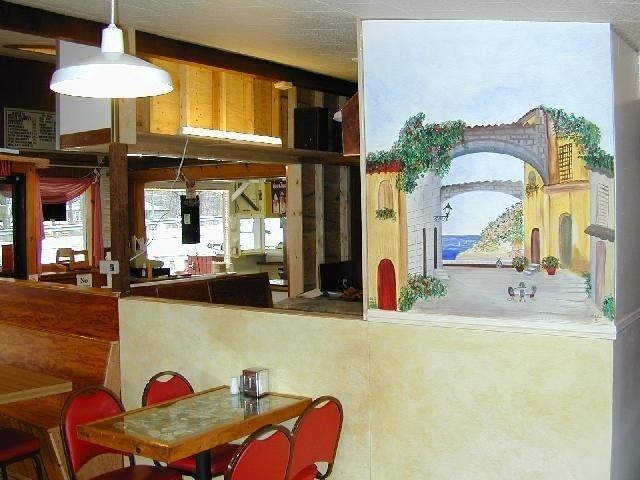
142, 369, 249, 480
505, 281, 538, 304
216, 422, 294, 480
57, 387, 186, 480
285, 394, 344, 480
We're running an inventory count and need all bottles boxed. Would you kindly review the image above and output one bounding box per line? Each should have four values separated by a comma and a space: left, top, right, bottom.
273, 192, 285, 214
141, 263, 146, 277
147, 263, 153, 281
230, 377, 240, 395
211, 260, 233, 274
231, 395, 238, 413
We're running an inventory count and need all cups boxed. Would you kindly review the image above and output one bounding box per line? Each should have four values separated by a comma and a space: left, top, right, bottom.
113, 292, 121, 299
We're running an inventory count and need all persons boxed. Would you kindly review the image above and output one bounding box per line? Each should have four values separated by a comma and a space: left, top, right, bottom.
496, 257, 502, 272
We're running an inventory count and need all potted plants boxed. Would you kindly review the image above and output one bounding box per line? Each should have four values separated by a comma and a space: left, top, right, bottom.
511, 256, 529, 272
537, 255, 561, 275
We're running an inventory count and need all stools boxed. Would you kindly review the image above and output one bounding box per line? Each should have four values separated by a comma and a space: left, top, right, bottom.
0, 425, 46, 480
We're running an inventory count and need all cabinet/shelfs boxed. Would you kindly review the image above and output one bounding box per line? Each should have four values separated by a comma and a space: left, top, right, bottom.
114, 55, 355, 156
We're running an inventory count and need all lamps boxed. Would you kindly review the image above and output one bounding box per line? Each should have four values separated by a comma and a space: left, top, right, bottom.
45, 0, 175, 101
432, 201, 453, 223
